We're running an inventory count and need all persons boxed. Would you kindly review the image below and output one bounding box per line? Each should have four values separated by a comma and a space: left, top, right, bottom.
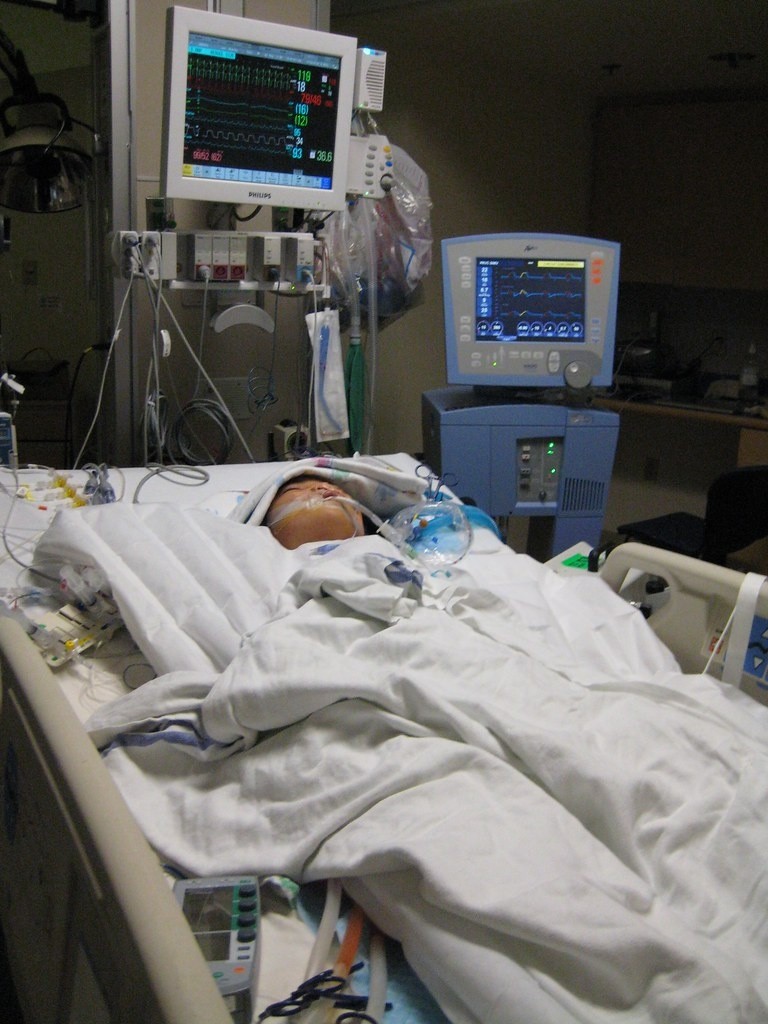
259, 474, 365, 550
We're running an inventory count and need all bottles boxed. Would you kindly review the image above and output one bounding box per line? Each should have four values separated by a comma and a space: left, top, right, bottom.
740, 339, 760, 400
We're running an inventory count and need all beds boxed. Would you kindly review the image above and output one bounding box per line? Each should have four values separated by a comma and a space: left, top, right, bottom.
0, 449, 768, 1024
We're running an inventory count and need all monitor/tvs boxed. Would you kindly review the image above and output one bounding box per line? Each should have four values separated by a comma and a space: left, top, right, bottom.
439, 231, 622, 389
158, 5, 358, 211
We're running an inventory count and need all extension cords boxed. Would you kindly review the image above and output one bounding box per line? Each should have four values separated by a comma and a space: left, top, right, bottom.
118, 229, 161, 279
